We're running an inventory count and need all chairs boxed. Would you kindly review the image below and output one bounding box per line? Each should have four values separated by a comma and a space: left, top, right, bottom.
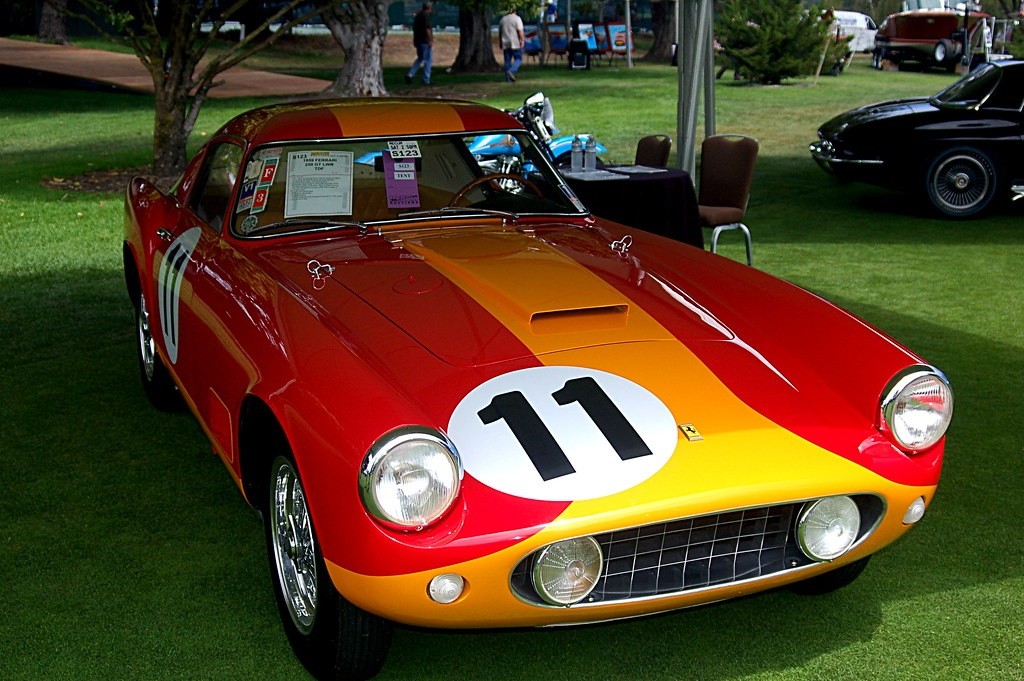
567, 39, 591, 70
695, 134, 760, 269
635, 134, 672, 169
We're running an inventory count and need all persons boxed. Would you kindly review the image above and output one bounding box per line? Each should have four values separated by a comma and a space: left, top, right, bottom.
498, 8, 526, 82
545, 1, 556, 24
404, 0, 434, 85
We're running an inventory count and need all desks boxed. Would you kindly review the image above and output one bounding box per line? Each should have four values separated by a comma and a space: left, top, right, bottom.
527, 163, 704, 250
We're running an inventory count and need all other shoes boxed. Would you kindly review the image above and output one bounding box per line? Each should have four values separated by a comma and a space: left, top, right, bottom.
404, 75, 411, 84
508, 71, 516, 81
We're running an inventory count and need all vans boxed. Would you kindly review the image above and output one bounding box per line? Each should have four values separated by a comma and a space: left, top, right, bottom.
875, 8, 991, 64
833, 10, 879, 53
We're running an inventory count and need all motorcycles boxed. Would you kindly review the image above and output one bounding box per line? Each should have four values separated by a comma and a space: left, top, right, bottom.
354, 92, 607, 197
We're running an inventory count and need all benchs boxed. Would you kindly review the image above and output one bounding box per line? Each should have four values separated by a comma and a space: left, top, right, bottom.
230, 178, 472, 236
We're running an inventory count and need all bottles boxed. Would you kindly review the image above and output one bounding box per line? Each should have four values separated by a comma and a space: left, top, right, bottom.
571, 135, 582, 172
585, 135, 596, 171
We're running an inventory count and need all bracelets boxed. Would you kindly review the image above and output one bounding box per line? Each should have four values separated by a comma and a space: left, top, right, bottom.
521, 37, 525, 41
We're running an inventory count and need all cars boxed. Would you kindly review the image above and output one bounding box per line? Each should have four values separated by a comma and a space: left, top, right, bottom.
809, 59, 1024, 219
122, 96, 956, 679
